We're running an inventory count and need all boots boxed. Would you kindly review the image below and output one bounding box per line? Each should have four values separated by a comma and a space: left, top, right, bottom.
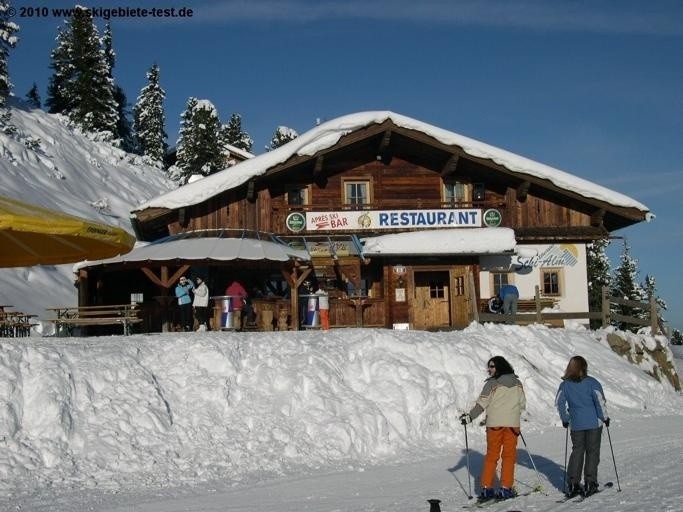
196, 325, 205, 332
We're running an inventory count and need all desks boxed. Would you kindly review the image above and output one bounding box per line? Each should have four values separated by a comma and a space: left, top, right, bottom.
46, 304, 141, 336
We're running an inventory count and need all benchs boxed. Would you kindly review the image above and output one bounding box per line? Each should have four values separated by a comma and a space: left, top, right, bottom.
46, 317, 144, 336
0, 305, 40, 337
480, 298, 555, 315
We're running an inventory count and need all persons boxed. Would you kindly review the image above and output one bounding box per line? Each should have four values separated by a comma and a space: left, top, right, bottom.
315, 282, 330, 330
225, 279, 257, 326
555, 355, 611, 497
500, 284, 519, 314
191, 277, 209, 332
175, 275, 194, 332
459, 356, 526, 499
488, 294, 503, 314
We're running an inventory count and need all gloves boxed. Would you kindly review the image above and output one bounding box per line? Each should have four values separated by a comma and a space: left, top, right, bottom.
459, 413, 472, 425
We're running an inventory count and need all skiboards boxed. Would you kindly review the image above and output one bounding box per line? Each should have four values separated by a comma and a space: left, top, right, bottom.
556, 482, 613, 503
463, 485, 542, 509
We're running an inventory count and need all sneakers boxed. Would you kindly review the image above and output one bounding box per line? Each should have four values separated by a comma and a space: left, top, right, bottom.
498, 487, 517, 500
584, 480, 598, 496
480, 487, 493, 498
566, 484, 582, 497
